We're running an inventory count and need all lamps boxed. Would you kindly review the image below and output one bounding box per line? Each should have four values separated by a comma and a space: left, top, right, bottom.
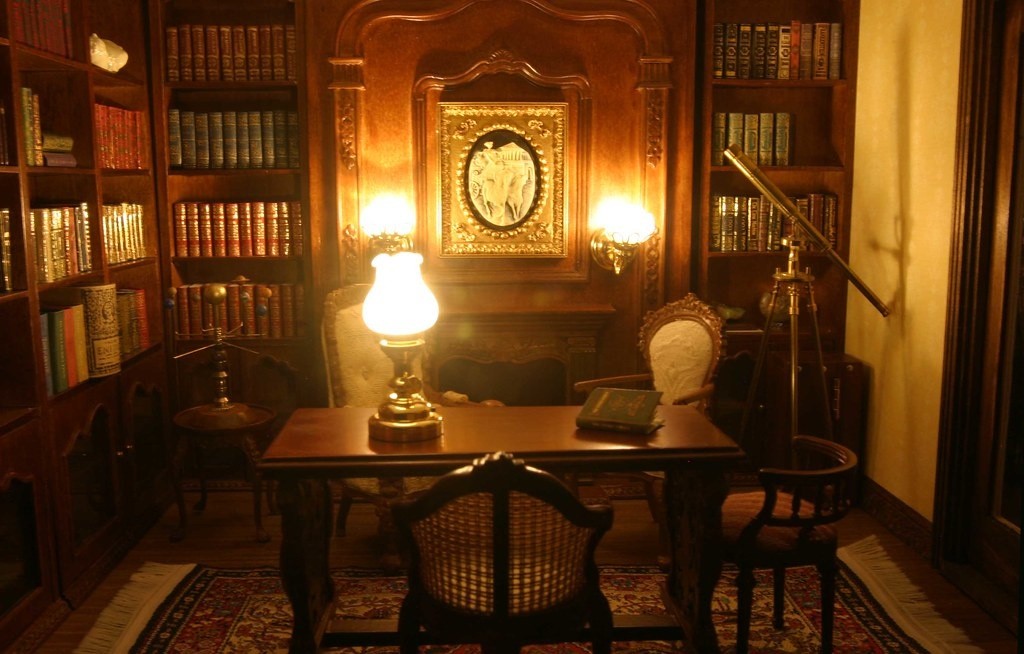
359, 191, 416, 253
362, 252, 443, 443
588, 196, 657, 275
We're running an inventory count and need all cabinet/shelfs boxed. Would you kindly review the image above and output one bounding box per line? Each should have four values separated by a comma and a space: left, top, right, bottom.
148, 0, 323, 491
700, 0, 862, 487
0, 0, 182, 654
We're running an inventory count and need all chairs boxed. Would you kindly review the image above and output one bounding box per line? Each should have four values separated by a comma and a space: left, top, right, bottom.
322, 283, 508, 554
718, 436, 858, 654
573, 292, 728, 419
389, 453, 615, 654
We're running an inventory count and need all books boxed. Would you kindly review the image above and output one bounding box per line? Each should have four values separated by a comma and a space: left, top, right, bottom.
714, 20, 841, 82
576, 386, 667, 435
0, 0, 303, 170
710, 194, 838, 253
1, 201, 307, 395
712, 112, 790, 166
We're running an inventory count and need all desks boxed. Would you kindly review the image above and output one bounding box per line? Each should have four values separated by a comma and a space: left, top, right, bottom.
428, 283, 617, 407
251, 407, 747, 654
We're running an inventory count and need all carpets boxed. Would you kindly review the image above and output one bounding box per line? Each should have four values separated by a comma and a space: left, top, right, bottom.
77, 534, 981, 654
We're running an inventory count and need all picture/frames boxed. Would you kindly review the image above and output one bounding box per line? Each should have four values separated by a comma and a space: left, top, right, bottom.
436, 100, 569, 259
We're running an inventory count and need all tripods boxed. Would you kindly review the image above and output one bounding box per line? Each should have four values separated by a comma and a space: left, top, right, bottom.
732, 219, 834, 469
